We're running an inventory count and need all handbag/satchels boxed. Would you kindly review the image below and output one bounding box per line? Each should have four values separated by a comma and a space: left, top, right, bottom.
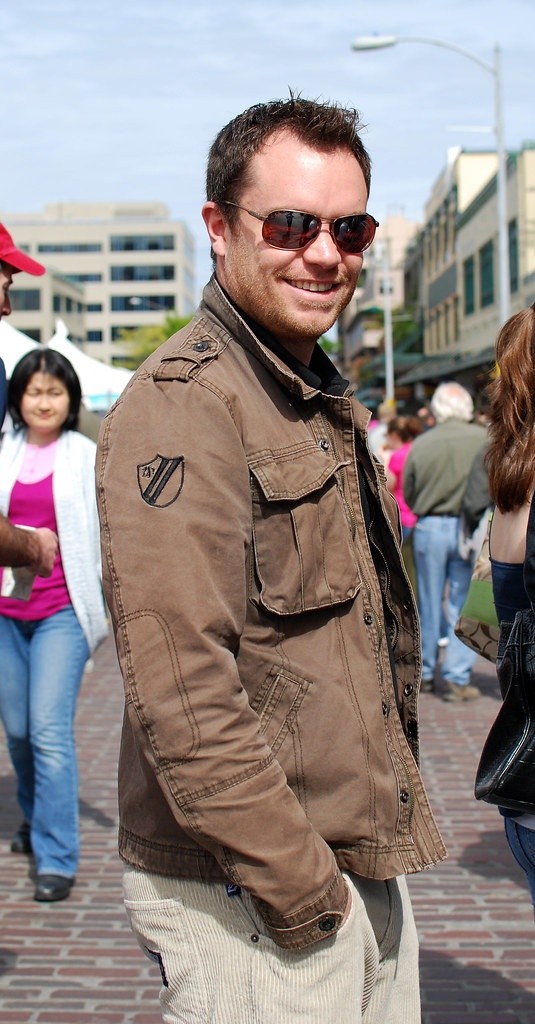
475, 492, 535, 814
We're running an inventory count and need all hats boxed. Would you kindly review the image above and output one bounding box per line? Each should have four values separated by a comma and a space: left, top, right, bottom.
0, 221, 47, 276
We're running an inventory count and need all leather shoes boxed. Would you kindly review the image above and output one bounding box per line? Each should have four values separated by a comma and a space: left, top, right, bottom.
35, 873, 76, 900
11, 821, 32, 852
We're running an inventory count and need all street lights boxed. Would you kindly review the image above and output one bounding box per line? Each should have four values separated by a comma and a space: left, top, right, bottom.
351, 34, 514, 339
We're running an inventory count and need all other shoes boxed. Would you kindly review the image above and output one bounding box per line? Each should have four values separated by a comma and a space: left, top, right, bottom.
443, 680, 484, 704
421, 680, 433, 691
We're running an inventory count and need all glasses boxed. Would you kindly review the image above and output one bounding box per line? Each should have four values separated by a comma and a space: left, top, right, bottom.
215, 197, 381, 253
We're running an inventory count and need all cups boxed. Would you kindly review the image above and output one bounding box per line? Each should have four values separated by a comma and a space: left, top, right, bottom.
2, 524, 40, 601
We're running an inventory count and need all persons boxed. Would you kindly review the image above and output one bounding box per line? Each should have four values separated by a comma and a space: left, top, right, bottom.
402, 380, 494, 700
1, 347, 109, 902
0, 221, 60, 579
474, 305, 535, 906
94, 93, 448, 1024
366, 400, 492, 548
